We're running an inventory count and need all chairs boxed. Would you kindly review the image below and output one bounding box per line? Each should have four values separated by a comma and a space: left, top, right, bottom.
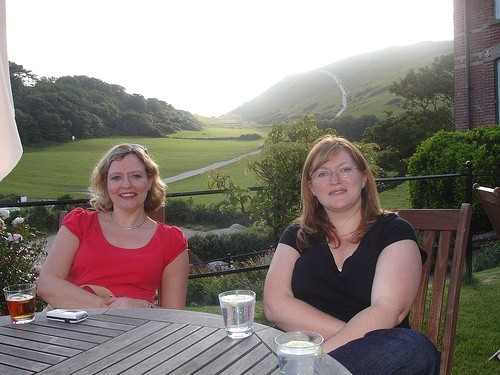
393, 203, 471, 375
473, 183, 500, 241
59, 202, 165, 235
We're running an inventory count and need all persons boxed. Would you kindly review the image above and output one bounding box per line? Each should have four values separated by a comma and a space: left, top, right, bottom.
38, 143, 189, 311
263, 136, 442, 375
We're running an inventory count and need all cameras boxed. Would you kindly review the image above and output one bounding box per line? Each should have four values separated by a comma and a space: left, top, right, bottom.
47, 308, 88, 323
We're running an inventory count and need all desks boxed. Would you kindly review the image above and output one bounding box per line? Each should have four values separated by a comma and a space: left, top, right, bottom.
0, 308, 355, 374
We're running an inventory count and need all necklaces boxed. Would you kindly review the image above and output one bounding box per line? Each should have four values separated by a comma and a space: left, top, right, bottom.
110, 211, 147, 229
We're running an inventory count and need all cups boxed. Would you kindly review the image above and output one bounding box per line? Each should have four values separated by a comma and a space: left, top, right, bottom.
218, 289, 256, 338
274, 331, 324, 375
3, 283, 36, 324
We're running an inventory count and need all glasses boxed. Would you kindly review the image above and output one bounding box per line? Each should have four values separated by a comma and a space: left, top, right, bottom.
310, 164, 362, 180
